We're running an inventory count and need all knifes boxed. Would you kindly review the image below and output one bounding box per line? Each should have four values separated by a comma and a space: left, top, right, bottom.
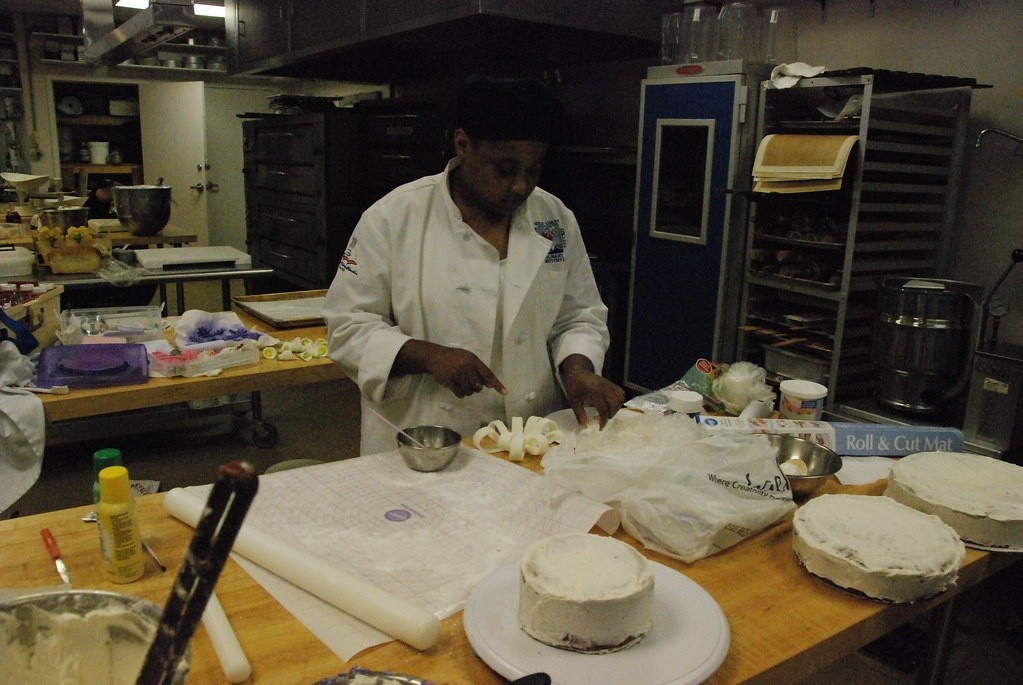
41, 528, 70, 583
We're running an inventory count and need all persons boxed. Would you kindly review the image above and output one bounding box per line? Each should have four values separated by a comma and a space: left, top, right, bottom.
325, 89, 628, 456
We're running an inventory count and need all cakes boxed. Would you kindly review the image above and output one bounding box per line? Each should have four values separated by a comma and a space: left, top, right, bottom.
519, 532, 655, 655
883, 449, 1023, 549
792, 492, 965, 604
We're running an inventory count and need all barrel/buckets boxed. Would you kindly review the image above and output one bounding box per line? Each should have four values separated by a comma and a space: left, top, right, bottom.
87, 141, 109, 164
873, 277, 982, 412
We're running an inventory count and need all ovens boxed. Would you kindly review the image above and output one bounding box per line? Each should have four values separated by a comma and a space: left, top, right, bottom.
243, 112, 434, 293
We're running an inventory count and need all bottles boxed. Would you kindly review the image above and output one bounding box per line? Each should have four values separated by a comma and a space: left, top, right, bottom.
93, 448, 145, 583
669, 391, 704, 419
0, 281, 56, 309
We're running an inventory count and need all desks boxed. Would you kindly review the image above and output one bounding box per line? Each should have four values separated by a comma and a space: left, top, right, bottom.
0, 217, 198, 318
30, 312, 357, 422
0, 415, 1023, 685
19, 263, 271, 317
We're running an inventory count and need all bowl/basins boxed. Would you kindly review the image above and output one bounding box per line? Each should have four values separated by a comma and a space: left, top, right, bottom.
752, 433, 842, 497
40, 206, 90, 232
0, 590, 190, 685
111, 184, 172, 236
396, 425, 463, 472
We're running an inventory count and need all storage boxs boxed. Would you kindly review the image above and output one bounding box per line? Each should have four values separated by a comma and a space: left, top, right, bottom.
0, 277, 63, 357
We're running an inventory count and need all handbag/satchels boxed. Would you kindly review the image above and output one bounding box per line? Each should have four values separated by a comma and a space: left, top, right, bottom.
548, 415, 791, 564
36, 342, 150, 390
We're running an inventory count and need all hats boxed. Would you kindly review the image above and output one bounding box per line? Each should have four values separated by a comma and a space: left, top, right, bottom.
447, 64, 563, 144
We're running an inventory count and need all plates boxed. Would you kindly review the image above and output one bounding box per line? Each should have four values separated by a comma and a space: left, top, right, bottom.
463, 558, 731, 685
